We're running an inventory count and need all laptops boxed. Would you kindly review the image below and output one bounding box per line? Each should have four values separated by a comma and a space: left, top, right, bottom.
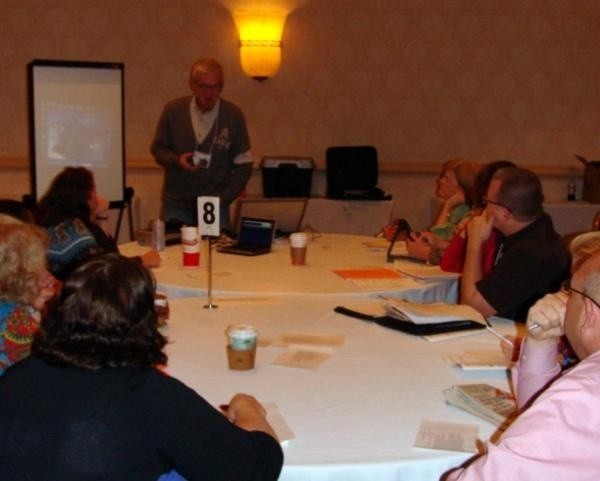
220, 217, 277, 257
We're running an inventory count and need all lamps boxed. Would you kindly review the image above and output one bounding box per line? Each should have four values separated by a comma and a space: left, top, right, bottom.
239, 45, 281, 83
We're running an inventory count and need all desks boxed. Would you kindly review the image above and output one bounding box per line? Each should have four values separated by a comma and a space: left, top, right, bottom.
228, 193, 395, 236
543, 201, 598, 238
143, 231, 459, 306
157, 298, 577, 481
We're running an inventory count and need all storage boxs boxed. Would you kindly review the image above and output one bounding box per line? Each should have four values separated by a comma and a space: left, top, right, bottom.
260, 155, 313, 198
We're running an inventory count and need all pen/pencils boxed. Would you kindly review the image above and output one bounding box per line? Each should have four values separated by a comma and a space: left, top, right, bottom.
219, 405, 230, 412
528, 324, 539, 330
487, 327, 513, 347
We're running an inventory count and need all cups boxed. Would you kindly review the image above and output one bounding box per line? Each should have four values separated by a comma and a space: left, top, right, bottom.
179, 224, 201, 270
225, 322, 259, 369
290, 232, 308, 266
152, 219, 165, 251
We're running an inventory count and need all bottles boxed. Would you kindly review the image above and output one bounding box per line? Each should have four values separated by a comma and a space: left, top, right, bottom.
567, 179, 576, 201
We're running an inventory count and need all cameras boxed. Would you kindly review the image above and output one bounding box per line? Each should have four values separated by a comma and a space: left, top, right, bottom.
192, 152, 212, 169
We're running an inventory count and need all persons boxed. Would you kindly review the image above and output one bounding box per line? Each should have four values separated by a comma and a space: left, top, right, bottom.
149, 57, 253, 246
0, 164, 284, 481
405, 158, 600, 481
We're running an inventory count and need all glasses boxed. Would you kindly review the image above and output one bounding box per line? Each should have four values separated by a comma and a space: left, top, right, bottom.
561, 279, 600, 309
481, 195, 512, 213
